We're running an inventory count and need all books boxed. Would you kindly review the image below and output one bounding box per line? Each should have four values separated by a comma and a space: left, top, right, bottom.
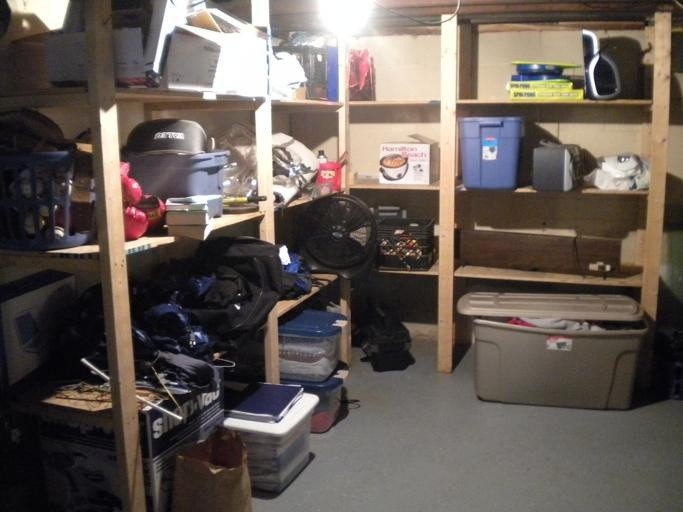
163, 195, 223, 243
222, 379, 304, 423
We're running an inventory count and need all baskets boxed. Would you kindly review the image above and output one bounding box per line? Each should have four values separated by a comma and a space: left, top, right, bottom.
1, 140, 98, 251
376, 217, 435, 271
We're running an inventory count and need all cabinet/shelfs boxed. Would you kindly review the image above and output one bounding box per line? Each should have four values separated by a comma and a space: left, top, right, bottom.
45, 6, 267, 99
0, 0, 671, 512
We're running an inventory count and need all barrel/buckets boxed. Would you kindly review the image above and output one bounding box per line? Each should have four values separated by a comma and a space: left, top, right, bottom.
581, 27, 647, 99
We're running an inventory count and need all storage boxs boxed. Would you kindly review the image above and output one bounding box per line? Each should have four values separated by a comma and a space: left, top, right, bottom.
456, 292, 650, 410
379, 134, 439, 186
120, 149, 228, 219
36, 359, 226, 512
251, 307, 349, 433
457, 117, 526, 191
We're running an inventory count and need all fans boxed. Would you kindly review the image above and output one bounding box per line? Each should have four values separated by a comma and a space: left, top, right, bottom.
300, 194, 378, 268
